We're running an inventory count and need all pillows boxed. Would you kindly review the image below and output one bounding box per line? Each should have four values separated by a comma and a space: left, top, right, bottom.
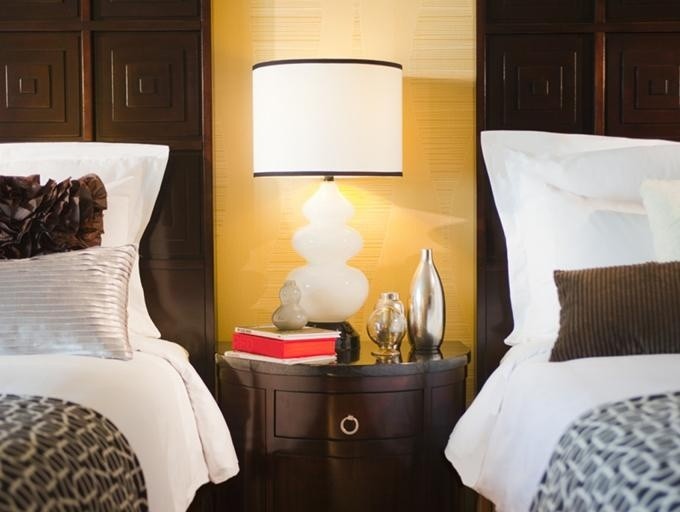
549, 260, 678, 361
480, 130, 679, 351
0, 141, 170, 362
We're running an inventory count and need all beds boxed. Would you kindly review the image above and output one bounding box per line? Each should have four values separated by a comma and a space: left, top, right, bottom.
441, 354, 680, 511
0, 338, 242, 512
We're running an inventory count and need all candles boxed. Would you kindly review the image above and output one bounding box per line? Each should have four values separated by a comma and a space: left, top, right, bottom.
381, 301, 392, 330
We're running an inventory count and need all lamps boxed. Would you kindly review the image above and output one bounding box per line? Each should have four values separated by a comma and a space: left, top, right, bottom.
251, 59, 404, 354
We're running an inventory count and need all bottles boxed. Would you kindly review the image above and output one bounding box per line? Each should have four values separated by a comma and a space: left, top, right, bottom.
380, 249, 446, 353
271, 280, 309, 330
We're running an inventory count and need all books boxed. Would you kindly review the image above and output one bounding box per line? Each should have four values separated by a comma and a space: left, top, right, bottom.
223, 350, 339, 365
232, 332, 337, 358
236, 324, 342, 341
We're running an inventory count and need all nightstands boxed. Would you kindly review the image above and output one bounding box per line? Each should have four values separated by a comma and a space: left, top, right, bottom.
212, 340, 471, 512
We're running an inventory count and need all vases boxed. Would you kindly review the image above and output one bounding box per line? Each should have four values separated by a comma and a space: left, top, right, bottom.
407, 248, 446, 355
